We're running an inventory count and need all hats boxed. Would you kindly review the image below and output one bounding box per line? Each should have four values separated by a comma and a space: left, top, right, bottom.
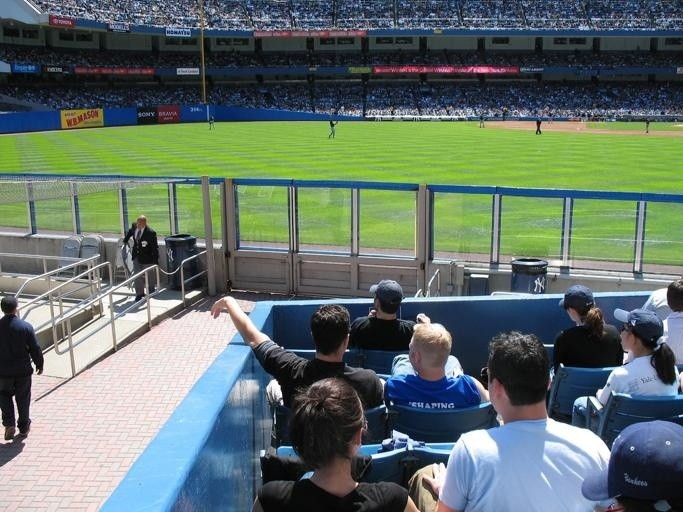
582, 419, 682, 501
370, 280, 403, 305
559, 285, 594, 308
2, 295, 18, 312
614, 308, 663, 340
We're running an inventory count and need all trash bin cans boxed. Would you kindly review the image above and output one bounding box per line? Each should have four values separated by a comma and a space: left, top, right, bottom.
510, 259, 548, 294
164, 233, 200, 291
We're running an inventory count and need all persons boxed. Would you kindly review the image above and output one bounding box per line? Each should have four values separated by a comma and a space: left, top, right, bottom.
552, 278, 683, 511
382, 321, 490, 408
124, 213, 159, 302
210, 296, 417, 512
0, 0, 683, 138
347, 280, 415, 353
0, 296, 44, 439
408, 331, 610, 511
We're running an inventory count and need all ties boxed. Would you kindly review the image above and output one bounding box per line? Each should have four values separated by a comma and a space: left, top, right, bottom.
137, 230, 141, 240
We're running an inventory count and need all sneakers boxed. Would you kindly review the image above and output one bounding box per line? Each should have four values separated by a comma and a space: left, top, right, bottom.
4, 426, 14, 440
135, 294, 144, 301
19, 428, 29, 436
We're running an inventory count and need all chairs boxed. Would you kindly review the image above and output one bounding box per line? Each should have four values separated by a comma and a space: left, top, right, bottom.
583, 389, 683, 451
274, 405, 388, 452
283, 348, 365, 375
366, 349, 411, 374
56, 234, 106, 281
544, 344, 556, 371
547, 362, 616, 418
388, 400, 500, 441
